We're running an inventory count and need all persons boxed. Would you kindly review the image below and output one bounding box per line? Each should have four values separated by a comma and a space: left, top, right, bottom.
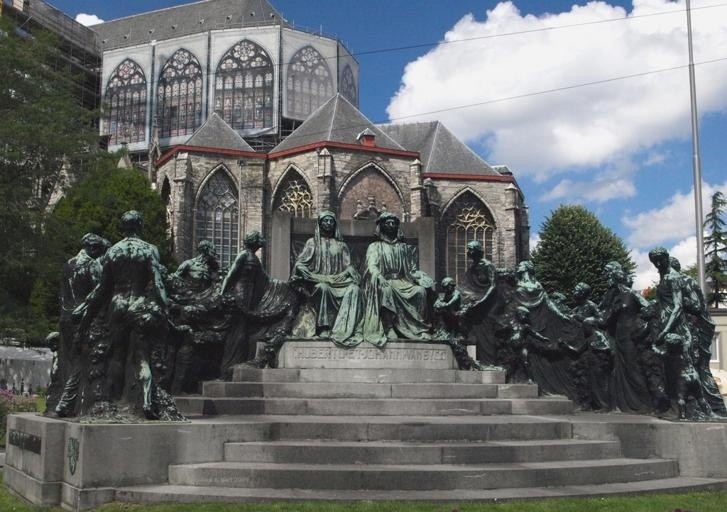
45, 208, 727, 423
